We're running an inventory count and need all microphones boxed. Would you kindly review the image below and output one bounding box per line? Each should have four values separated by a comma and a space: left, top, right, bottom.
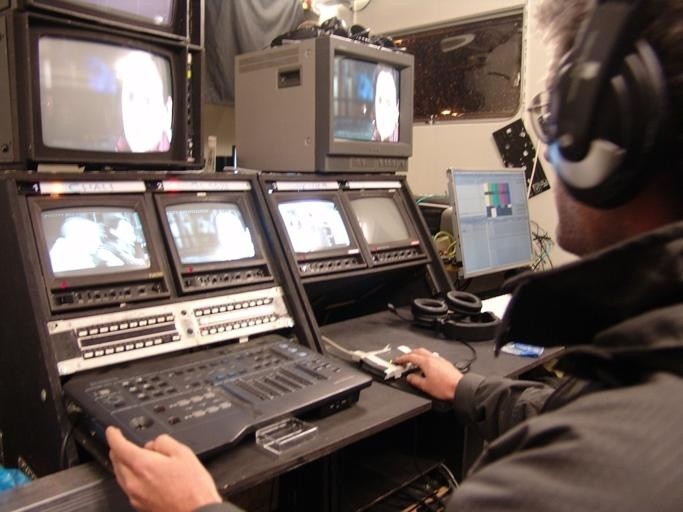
387, 302, 415, 323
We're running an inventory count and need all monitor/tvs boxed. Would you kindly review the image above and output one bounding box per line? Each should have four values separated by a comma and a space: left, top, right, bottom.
25, 192, 172, 313
152, 191, 274, 295
0, 0, 205, 49
339, 188, 427, 269
446, 167, 534, 281
0, 10, 204, 171
266, 190, 369, 278
235, 33, 414, 174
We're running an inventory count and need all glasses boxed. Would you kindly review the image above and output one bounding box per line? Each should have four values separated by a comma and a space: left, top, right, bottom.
528, 90, 557, 144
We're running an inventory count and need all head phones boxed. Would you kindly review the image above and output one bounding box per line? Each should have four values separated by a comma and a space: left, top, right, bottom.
411, 289, 503, 341
271, 17, 348, 46
546, 0, 667, 209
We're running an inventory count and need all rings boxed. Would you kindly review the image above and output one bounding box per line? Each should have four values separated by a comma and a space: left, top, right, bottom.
430, 350, 440, 358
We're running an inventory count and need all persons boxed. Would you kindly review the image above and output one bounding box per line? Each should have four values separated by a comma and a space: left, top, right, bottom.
371, 58, 401, 145
103, 1, 682, 511
97, 211, 146, 269
209, 206, 252, 261
109, 43, 174, 153
46, 216, 103, 273
